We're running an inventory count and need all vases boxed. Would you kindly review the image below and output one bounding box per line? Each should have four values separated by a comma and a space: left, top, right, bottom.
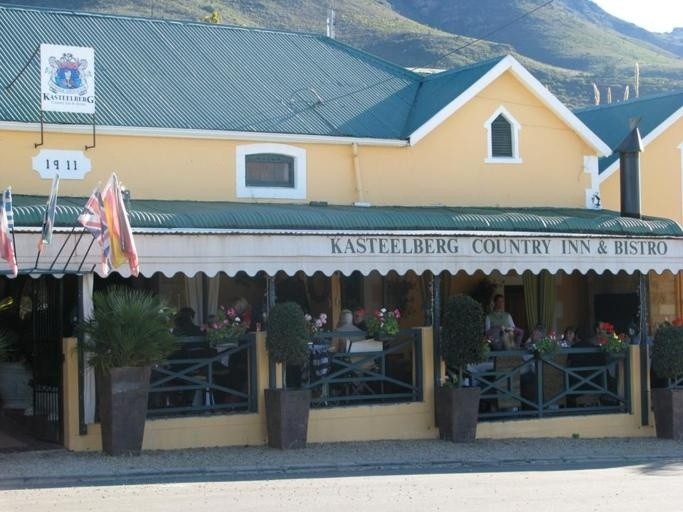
216, 342, 240, 368
307, 342, 329, 377
375, 329, 396, 342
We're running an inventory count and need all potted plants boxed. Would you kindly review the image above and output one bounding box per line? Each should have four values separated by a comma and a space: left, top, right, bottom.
651, 319, 683, 440
71, 283, 188, 458
437, 293, 488, 444
264, 300, 312, 450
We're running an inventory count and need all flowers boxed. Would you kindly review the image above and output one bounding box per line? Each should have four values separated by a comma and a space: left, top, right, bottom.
529, 329, 558, 353
304, 311, 328, 344
365, 308, 401, 336
593, 321, 627, 354
207, 303, 242, 342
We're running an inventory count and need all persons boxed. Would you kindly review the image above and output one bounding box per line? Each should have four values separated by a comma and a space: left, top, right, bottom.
486, 294, 516, 334
524, 321, 672, 344
149, 295, 255, 407
330, 307, 373, 387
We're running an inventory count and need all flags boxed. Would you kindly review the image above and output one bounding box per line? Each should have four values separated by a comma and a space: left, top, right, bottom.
0, 186, 18, 279
77, 173, 140, 278
37, 174, 60, 257
